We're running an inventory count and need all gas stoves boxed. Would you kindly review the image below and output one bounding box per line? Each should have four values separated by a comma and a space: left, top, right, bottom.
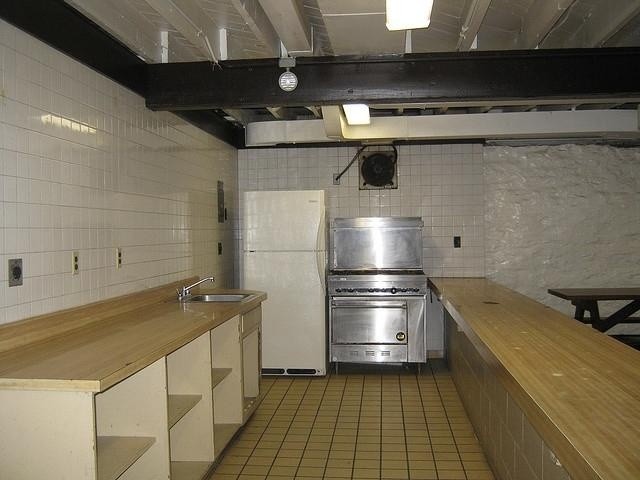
327, 266, 425, 296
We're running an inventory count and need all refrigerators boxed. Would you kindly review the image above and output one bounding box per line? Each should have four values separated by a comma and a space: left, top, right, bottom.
243, 188, 330, 378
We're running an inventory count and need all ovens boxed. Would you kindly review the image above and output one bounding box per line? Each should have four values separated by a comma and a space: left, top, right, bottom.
326, 294, 428, 378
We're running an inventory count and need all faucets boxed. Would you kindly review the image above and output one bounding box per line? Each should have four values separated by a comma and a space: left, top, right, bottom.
183, 277, 215, 295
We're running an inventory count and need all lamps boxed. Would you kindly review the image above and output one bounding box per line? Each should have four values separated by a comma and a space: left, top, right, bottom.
385, 0, 434, 32
342, 104, 371, 126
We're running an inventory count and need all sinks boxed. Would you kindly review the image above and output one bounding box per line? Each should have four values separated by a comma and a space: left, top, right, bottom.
178, 293, 256, 303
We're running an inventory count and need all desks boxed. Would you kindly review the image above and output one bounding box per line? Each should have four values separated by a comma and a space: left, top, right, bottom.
426, 277, 640, 480
547, 287, 640, 351
